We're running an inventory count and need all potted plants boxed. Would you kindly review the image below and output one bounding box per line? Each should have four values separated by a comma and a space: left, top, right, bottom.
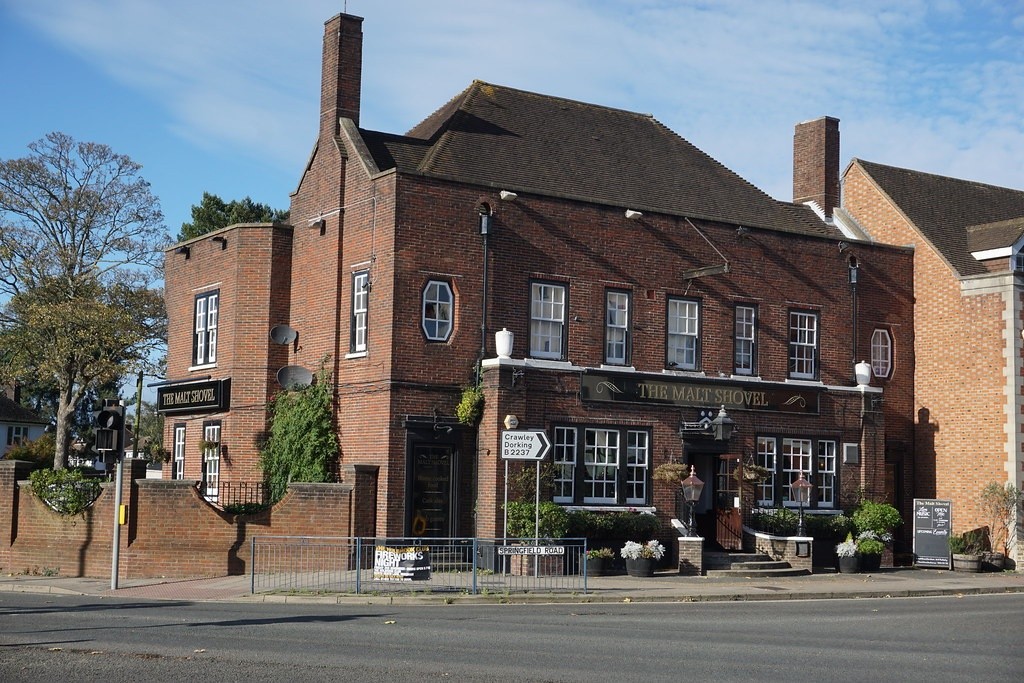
651, 459, 687, 485
857, 537, 884, 572
976, 482, 1023, 573
948, 529, 983, 573
198, 437, 218, 457
732, 462, 771, 483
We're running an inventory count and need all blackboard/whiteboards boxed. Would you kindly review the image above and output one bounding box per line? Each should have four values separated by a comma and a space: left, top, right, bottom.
912, 497, 955, 571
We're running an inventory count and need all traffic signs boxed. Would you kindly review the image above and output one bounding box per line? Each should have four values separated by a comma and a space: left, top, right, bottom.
499, 430, 553, 460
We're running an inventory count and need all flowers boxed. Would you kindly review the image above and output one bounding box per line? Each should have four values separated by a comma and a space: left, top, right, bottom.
576, 546, 616, 560
620, 539, 666, 560
833, 540, 859, 559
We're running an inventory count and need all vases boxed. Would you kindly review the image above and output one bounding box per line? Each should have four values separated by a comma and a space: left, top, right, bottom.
626, 558, 658, 577
579, 557, 612, 576
834, 557, 857, 573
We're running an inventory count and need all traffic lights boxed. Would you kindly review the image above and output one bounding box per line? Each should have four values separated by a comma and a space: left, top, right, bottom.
94, 398, 125, 464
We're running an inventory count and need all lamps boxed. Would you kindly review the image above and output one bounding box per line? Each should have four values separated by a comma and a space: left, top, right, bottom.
838, 241, 849, 252
707, 404, 734, 443
212, 237, 228, 251
625, 210, 642, 219
736, 225, 749, 235
680, 465, 705, 537
499, 191, 517, 201
175, 247, 190, 259
307, 218, 322, 229
791, 469, 814, 537
